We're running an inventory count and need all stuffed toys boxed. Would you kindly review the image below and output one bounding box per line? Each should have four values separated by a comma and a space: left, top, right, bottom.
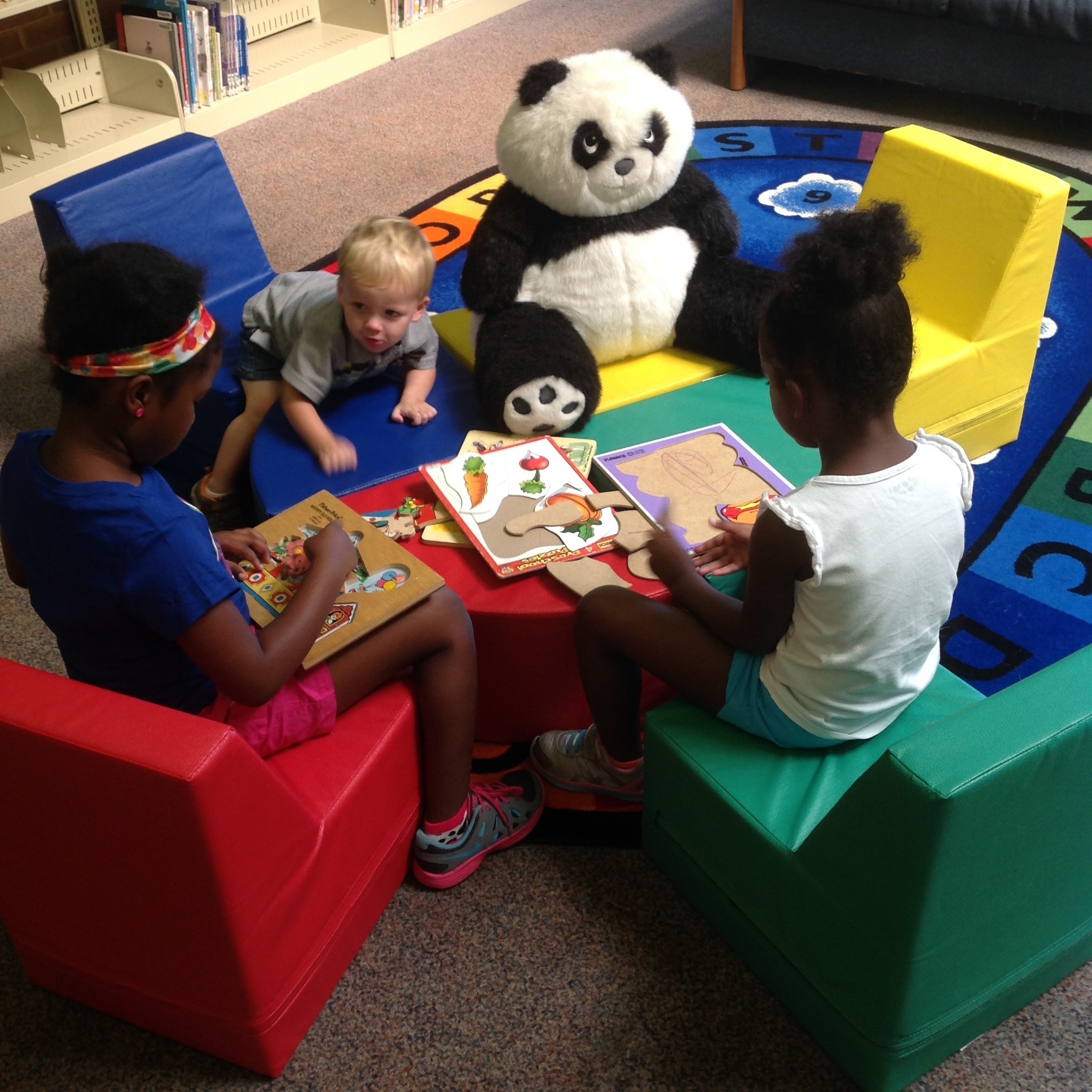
460, 49, 780, 438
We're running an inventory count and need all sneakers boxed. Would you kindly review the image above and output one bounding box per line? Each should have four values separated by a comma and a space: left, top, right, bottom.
413, 769, 546, 889
531, 732, 645, 797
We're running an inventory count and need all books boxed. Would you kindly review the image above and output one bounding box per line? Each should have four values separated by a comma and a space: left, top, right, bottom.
108, 1, 253, 114
390, 0, 444, 31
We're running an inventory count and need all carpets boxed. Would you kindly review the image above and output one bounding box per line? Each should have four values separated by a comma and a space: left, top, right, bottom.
246, 116, 1092, 811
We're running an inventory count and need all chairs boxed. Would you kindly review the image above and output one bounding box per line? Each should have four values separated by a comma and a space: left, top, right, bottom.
639, 641, 1092, 1090
24, 125, 307, 422
849, 116, 1074, 464
0, 656, 423, 1079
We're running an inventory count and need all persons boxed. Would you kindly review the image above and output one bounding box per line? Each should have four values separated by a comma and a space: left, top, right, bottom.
184, 215, 441, 536
0, 233, 546, 892
528, 200, 977, 804
